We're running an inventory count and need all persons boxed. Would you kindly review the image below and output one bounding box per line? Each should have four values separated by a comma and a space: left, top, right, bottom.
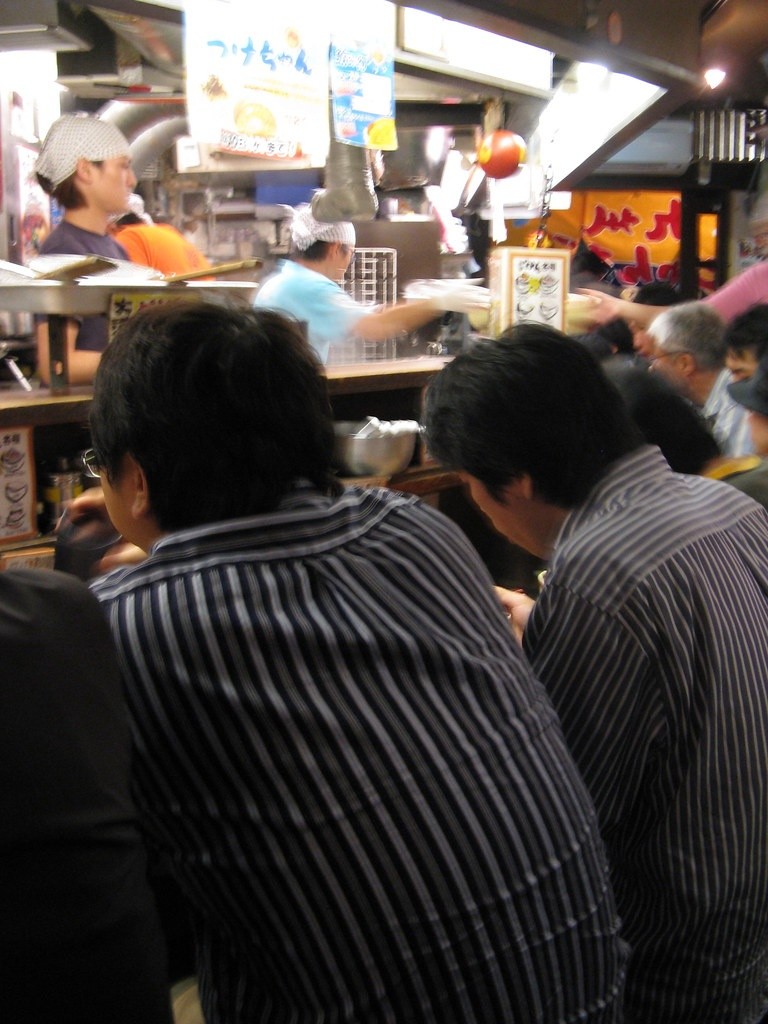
254, 203, 491, 366
54, 300, 631, 1024
575, 259, 768, 515
106, 192, 216, 282
34, 114, 138, 491
0, 565, 174, 1024
421, 322, 768, 1023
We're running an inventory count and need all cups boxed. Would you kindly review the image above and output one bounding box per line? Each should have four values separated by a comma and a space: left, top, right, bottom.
51, 512, 123, 584
40, 472, 83, 532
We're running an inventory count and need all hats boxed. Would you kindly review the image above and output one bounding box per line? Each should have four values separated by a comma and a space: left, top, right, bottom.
727, 356, 768, 417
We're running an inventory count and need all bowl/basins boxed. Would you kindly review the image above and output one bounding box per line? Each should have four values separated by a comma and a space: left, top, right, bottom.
332, 420, 418, 477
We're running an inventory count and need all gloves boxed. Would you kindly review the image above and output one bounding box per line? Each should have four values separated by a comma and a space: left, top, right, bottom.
425, 276, 491, 315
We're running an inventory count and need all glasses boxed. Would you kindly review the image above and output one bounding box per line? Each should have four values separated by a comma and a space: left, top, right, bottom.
647, 349, 684, 366
81, 447, 105, 477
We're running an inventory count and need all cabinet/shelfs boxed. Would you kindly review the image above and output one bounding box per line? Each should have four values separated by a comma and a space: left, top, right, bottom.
0, 355, 472, 582
